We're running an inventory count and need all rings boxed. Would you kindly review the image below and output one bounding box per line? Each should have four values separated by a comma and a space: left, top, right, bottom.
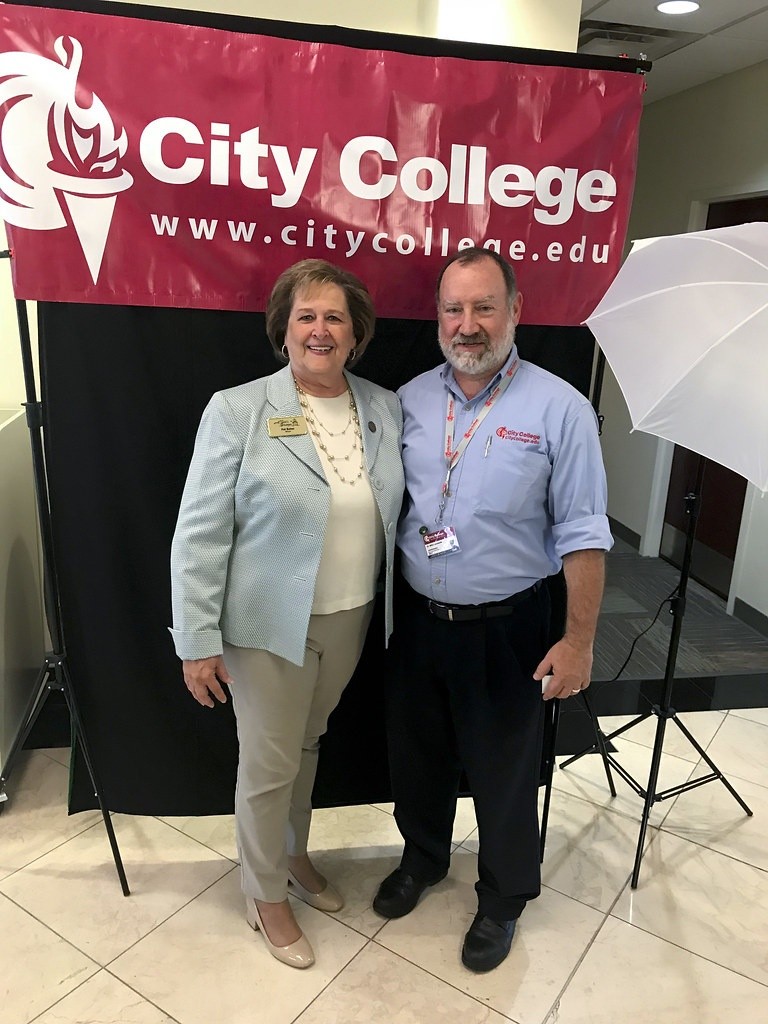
572, 689, 581, 693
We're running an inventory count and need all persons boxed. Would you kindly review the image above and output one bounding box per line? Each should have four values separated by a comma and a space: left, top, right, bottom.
372, 247, 616, 973
168, 258, 406, 968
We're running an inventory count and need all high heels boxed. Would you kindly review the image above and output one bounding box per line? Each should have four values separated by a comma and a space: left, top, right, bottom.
246, 897, 316, 970
288, 868, 345, 913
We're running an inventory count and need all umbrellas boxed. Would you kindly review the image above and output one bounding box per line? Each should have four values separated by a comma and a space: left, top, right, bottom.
580, 221, 768, 493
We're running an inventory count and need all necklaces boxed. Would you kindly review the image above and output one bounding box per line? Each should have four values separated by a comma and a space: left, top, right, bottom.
294, 380, 364, 484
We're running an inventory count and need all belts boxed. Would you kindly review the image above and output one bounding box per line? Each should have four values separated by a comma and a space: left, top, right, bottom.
425, 580, 543, 622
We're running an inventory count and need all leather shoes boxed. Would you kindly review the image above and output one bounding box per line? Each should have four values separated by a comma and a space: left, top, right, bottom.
462, 911, 518, 975
373, 866, 449, 919
